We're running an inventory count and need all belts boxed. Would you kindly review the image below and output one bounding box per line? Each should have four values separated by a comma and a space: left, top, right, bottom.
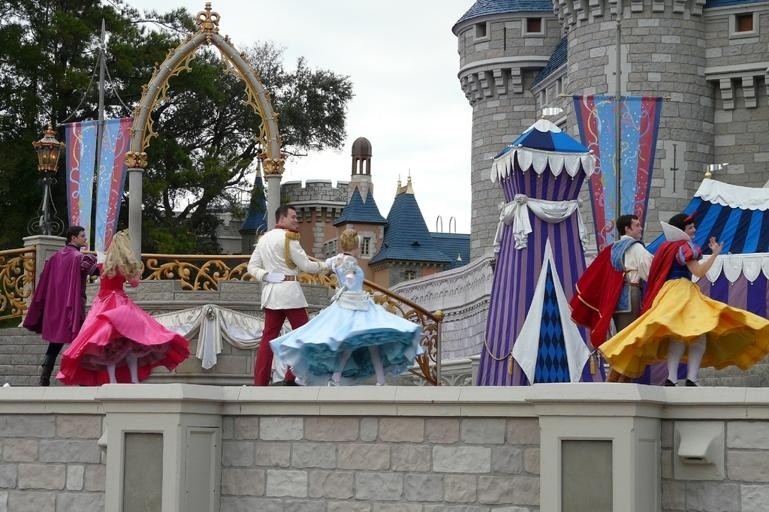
284, 275, 298, 281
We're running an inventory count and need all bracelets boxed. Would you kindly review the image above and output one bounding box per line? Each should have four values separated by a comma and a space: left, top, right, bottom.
711, 252, 718, 259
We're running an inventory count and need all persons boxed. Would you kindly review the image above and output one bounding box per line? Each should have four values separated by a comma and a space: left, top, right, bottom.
569, 213, 656, 382
268, 228, 427, 387
594, 211, 769, 387
21, 224, 102, 387
246, 204, 336, 387
55, 228, 192, 386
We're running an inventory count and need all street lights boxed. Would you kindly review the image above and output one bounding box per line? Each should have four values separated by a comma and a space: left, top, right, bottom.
29, 122, 65, 236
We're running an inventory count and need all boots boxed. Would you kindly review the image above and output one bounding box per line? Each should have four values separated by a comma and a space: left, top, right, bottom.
39, 354, 57, 386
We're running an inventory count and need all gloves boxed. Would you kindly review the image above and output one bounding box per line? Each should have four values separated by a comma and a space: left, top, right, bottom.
262, 273, 285, 283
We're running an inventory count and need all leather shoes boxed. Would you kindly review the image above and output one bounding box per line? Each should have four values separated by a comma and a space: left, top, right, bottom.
283, 380, 300, 385
665, 379, 678, 386
685, 379, 699, 386
327, 378, 341, 386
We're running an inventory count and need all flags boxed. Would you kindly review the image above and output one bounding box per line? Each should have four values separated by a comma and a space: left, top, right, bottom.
64, 117, 131, 256
573, 93, 663, 315
